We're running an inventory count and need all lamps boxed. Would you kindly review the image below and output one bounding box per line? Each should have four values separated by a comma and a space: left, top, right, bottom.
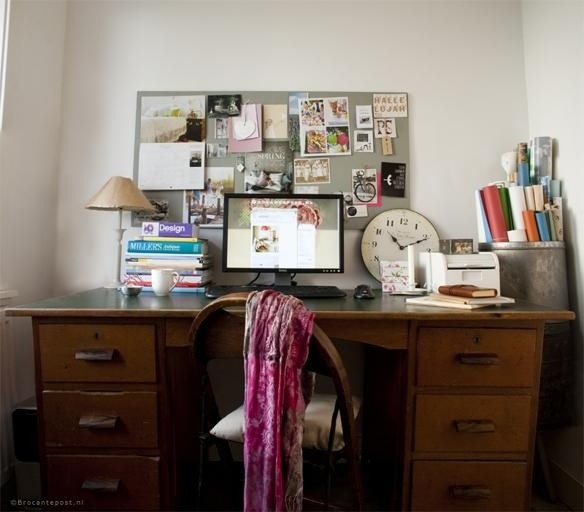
85, 176, 156, 288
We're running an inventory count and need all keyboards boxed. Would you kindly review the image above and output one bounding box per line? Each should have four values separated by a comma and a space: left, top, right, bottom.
205, 285, 346, 299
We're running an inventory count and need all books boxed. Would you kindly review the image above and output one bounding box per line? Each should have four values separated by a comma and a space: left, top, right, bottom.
437, 283, 497, 299
405, 294, 498, 310
431, 290, 516, 306
119, 217, 217, 295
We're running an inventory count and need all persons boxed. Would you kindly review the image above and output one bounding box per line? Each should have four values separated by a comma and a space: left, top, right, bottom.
377, 118, 392, 135
206, 177, 213, 196
190, 191, 201, 210
214, 179, 223, 197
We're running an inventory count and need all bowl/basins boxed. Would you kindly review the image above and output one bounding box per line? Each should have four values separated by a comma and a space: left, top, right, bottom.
120, 286, 142, 296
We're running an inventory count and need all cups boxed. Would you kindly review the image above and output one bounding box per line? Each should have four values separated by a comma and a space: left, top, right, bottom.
150, 268, 179, 297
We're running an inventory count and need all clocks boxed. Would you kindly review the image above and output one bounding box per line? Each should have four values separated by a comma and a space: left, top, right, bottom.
360, 208, 441, 284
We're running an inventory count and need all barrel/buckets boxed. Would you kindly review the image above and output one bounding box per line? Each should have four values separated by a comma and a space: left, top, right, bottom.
536, 329, 577, 432
479, 240, 572, 336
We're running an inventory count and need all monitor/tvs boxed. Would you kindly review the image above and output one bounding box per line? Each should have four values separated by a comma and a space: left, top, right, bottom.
222, 193, 344, 287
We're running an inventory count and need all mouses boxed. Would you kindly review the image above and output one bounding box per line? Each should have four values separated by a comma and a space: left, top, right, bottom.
354, 285, 375, 299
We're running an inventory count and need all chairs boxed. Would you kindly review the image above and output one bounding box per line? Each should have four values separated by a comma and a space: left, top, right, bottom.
185, 293, 369, 511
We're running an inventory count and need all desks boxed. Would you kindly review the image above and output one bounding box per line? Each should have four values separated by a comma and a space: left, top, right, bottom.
6, 289, 575, 511
